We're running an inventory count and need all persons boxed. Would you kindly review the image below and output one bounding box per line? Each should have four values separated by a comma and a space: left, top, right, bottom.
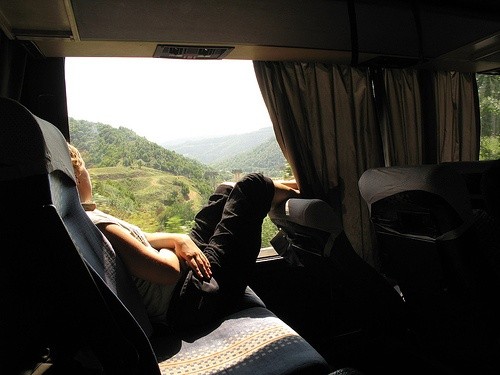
67, 141, 301, 332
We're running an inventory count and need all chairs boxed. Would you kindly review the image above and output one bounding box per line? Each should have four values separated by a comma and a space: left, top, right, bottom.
0, 92, 356, 375
266, 159, 500, 375
